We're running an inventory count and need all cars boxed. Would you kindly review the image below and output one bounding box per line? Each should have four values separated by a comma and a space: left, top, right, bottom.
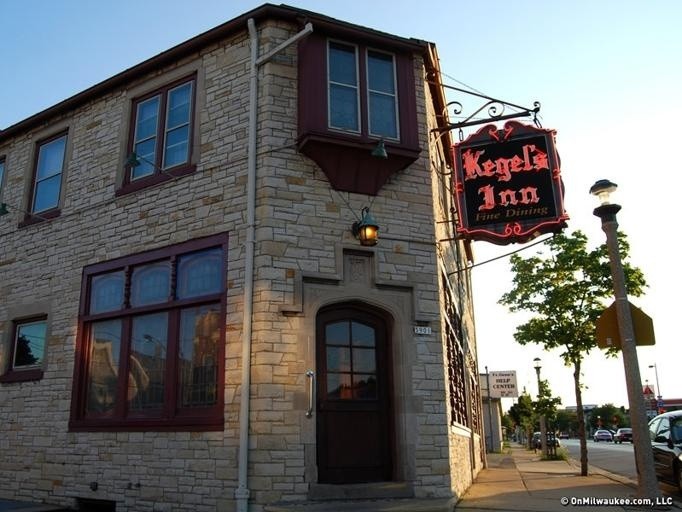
649, 409, 682, 492
560, 431, 569, 439
592, 430, 613, 442
535, 431, 558, 447
613, 428, 633, 443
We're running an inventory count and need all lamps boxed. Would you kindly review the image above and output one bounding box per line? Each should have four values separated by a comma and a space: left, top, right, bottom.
352, 206, 379, 246
372, 139, 387, 160
122, 152, 179, 179
0, 202, 49, 222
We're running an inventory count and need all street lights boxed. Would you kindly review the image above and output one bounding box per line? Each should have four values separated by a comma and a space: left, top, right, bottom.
590, 180, 662, 501
648, 364, 661, 397
533, 358, 550, 460
